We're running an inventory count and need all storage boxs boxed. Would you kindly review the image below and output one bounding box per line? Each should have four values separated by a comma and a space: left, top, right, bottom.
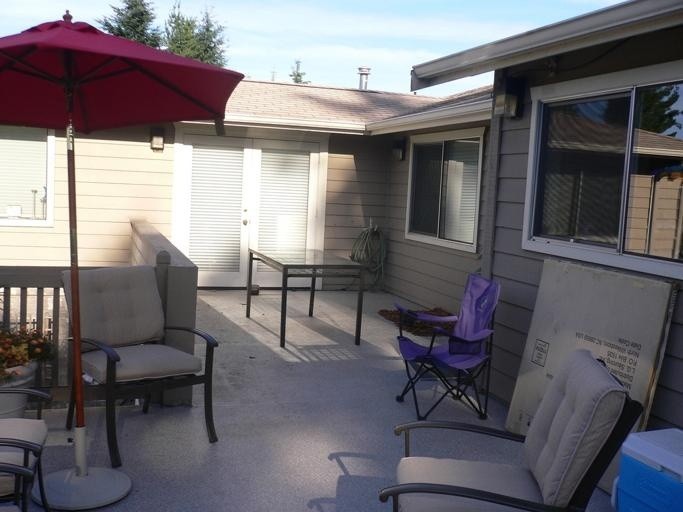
613, 429, 682, 512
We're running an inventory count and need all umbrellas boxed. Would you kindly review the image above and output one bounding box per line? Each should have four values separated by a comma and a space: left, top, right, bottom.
0, 10, 245, 426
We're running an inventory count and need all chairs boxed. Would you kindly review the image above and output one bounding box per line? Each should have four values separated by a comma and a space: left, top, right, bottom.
379, 347, 642, 512
56, 262, 218, 468
394, 268, 500, 424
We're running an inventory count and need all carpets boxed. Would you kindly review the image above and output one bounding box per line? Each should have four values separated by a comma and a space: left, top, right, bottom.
377, 300, 456, 338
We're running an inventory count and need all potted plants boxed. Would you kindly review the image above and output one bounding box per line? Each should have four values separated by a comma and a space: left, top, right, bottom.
352, 217, 389, 287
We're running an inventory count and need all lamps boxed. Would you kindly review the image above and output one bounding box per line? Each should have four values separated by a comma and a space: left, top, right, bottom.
148, 126, 166, 152
392, 137, 406, 161
489, 71, 525, 121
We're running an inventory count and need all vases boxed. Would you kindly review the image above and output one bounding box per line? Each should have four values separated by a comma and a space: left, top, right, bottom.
0, 360, 38, 419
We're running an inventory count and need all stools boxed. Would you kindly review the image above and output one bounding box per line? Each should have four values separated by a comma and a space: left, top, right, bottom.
0, 387, 54, 512
1, 462, 34, 512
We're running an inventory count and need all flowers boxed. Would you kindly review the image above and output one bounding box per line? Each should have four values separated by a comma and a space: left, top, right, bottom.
0, 322, 50, 383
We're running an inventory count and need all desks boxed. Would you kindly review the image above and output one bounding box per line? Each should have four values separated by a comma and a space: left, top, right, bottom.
244, 241, 369, 348
